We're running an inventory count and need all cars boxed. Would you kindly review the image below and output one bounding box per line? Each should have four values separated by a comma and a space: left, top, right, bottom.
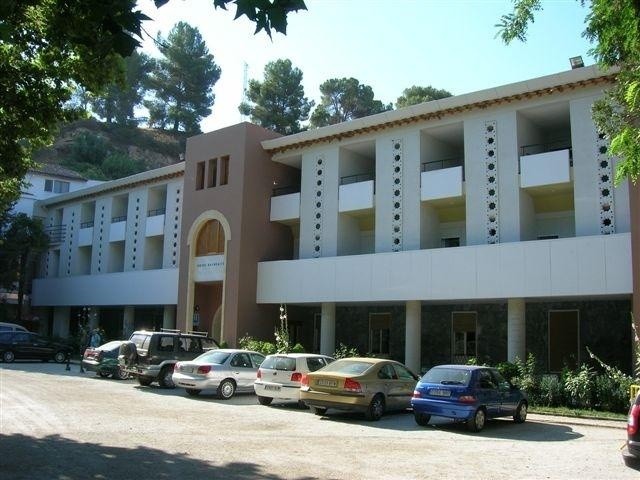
82, 338, 135, 380
408, 362, 530, 432
253, 353, 339, 411
621, 389, 640, 472
299, 355, 425, 421
170, 347, 269, 402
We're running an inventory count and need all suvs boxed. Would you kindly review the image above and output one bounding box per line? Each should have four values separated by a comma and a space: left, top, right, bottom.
116, 325, 227, 391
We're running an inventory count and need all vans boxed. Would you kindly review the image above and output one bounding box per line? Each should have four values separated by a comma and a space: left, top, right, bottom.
1, 322, 31, 334
0, 331, 73, 368
